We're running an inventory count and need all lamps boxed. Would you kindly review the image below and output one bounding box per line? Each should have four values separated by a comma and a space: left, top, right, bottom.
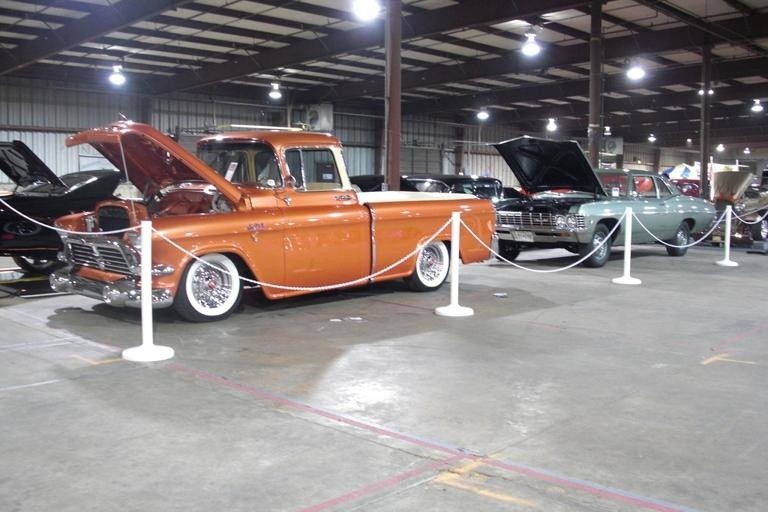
522, 28, 539, 55
109, 62, 125, 84
269, 80, 282, 98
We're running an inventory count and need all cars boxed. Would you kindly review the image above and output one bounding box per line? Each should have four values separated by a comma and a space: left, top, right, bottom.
489, 135, 718, 269
346, 134, 768, 245
0, 138, 121, 276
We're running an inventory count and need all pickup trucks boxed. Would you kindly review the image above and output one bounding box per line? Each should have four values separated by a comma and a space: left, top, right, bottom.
53, 116, 494, 323
